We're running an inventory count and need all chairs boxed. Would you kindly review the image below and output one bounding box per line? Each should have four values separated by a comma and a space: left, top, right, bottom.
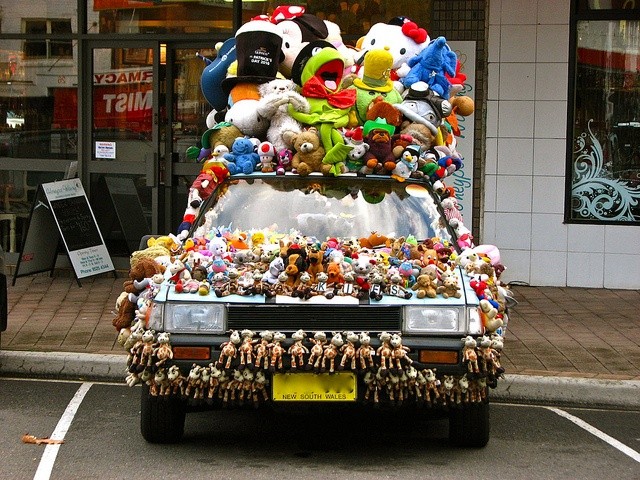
0, 181, 33, 254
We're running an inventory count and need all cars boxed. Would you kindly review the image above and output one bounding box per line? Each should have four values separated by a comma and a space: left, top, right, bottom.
133, 168, 497, 449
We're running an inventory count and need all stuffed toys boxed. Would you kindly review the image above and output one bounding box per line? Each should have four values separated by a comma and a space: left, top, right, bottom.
117, 328, 185, 395
169, 223, 462, 300
185, 329, 505, 406
176, 5, 474, 248
186, 329, 505, 405
112, 233, 182, 332
175, 4, 474, 248
455, 244, 508, 328
